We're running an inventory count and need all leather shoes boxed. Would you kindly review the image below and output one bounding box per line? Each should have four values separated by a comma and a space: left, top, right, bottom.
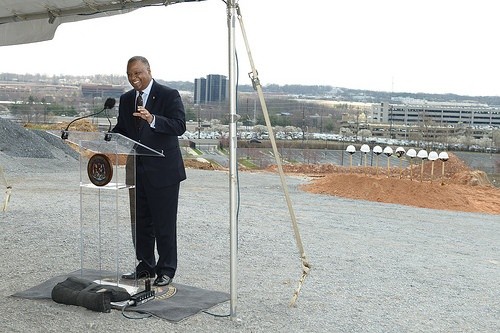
122, 271, 155, 279
154, 274, 172, 286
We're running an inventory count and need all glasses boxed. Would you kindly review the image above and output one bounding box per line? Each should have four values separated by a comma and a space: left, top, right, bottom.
127, 68, 148, 77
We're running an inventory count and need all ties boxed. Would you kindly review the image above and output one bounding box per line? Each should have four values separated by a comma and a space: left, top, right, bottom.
135, 91, 143, 138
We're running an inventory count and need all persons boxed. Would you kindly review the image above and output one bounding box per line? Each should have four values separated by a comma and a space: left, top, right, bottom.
109, 56, 187, 286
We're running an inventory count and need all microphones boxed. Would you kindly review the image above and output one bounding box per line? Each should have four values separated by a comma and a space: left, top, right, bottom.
65, 97, 112, 132
104, 98, 116, 132
